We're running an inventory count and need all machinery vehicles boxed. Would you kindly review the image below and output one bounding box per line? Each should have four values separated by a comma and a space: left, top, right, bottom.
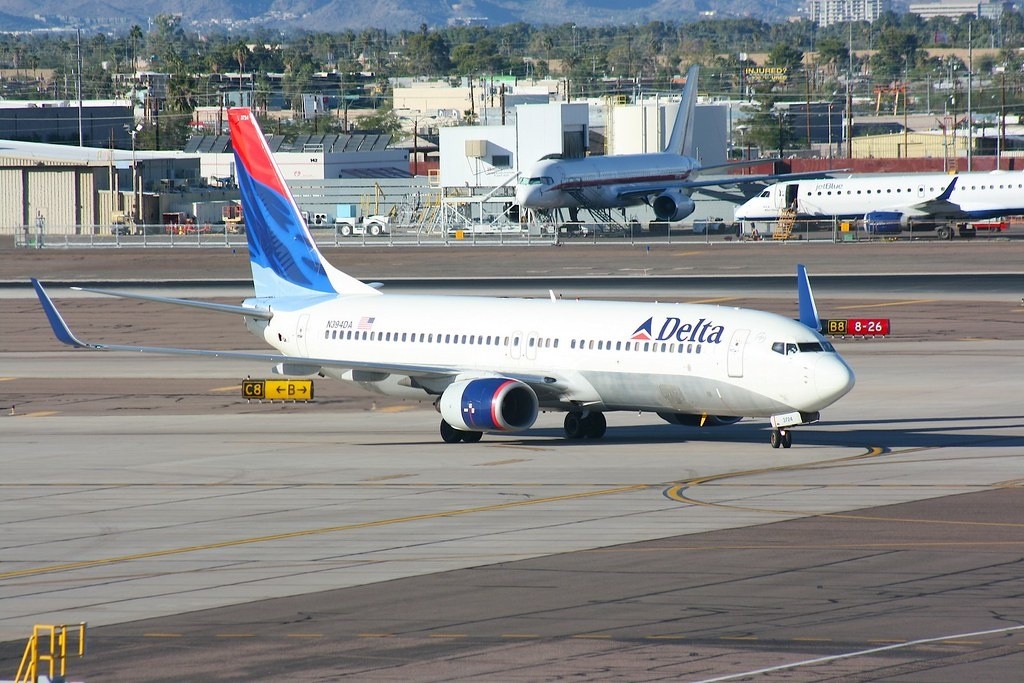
333, 211, 394, 236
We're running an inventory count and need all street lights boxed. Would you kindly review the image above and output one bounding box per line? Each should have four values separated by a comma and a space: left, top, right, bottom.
123, 123, 144, 220
938, 58, 949, 85
827, 97, 838, 171
930, 112, 948, 173
933, 69, 941, 90
737, 125, 750, 162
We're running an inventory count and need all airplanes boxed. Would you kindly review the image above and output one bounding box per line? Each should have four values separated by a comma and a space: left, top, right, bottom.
731, 167, 1024, 241
28, 105, 855, 444
445, 64, 853, 234
